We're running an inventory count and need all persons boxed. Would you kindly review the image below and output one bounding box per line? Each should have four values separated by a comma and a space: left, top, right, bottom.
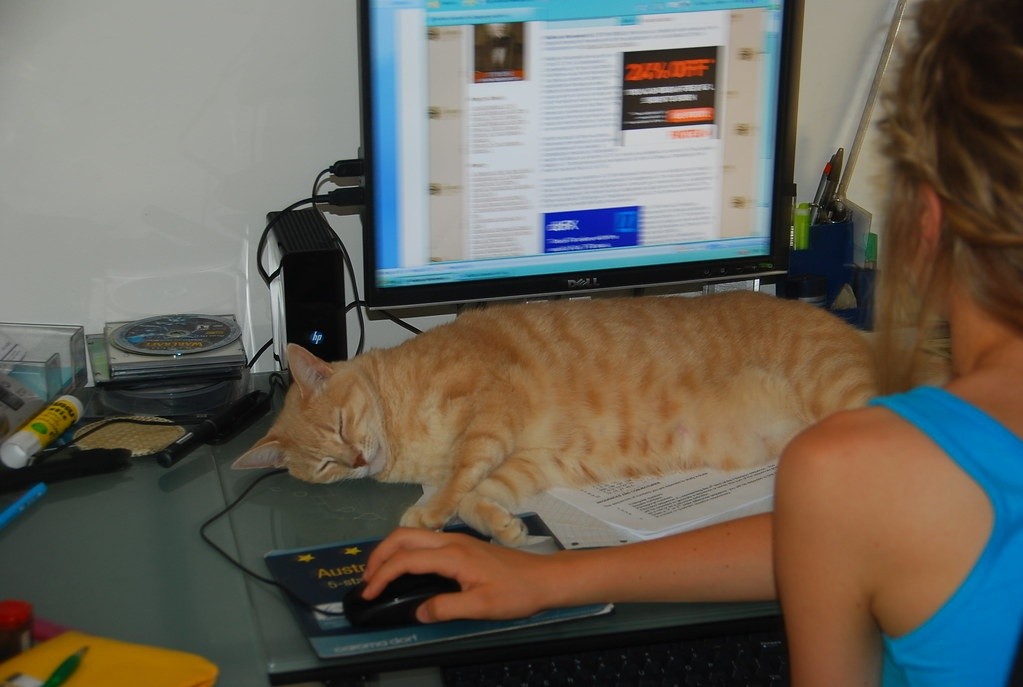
362, 0, 1023, 687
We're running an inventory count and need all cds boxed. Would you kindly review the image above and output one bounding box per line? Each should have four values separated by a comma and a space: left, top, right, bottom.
113, 313, 241, 355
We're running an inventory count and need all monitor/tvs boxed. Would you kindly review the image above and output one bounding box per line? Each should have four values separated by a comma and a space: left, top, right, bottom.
353, 0, 807, 320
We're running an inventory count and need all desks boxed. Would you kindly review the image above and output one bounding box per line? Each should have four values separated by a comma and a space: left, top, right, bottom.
0, 296, 883, 687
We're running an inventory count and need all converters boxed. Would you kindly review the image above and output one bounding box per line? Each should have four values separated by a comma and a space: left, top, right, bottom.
4, 443, 120, 489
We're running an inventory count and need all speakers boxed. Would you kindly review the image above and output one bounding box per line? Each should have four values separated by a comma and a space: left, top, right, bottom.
264, 210, 348, 395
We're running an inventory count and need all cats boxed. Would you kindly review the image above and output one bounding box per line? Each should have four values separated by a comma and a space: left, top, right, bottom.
229, 288, 883, 548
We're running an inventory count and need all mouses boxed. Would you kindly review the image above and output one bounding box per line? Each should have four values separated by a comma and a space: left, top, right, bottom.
344, 573, 462, 629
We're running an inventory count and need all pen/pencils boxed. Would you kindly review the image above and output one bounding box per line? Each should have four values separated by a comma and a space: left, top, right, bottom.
1, 483, 49, 529
32, 645, 88, 687
786, 146, 845, 250
